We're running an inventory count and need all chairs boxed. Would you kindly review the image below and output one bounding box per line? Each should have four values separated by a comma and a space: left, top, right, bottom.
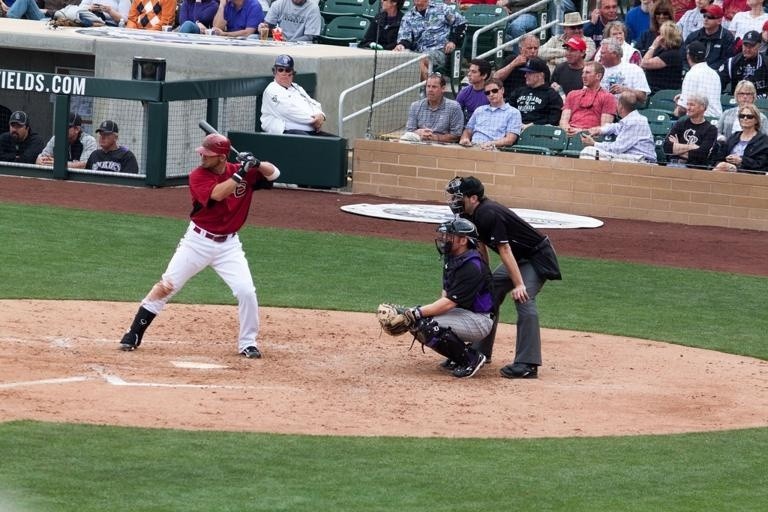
311, 17, 371, 47
320, 0, 371, 18
500, 125, 567, 156
364, 0, 766, 143
559, 129, 616, 159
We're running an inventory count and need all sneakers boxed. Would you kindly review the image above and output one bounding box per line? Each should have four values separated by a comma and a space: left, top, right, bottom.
499, 363, 538, 378
440, 351, 486, 378
120, 330, 142, 350
241, 346, 261, 358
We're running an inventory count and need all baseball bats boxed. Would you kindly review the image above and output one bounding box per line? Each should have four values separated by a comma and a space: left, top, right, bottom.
198, 119, 256, 170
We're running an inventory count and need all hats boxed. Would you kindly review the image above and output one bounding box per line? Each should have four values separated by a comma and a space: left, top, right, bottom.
9, 110, 29, 127
700, 4, 724, 18
742, 29, 761, 45
557, 11, 591, 27
68, 111, 82, 127
95, 119, 118, 133
562, 36, 587, 52
520, 56, 547, 72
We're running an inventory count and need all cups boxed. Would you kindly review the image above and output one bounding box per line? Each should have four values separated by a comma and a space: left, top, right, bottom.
161, 26, 172, 32
204, 29, 215, 36
349, 43, 358, 48
260, 28, 269, 40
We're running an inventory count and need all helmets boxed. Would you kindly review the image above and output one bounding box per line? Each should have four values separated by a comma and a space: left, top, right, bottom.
435, 217, 480, 261
274, 54, 294, 67
445, 177, 484, 214
195, 133, 231, 159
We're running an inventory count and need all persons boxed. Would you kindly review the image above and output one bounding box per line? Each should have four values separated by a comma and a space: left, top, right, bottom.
263, 56, 340, 137
446, 176, 563, 377
391, 219, 495, 379
119, 133, 280, 358
356, 0, 766, 175
0, 0, 322, 42
0, 110, 139, 172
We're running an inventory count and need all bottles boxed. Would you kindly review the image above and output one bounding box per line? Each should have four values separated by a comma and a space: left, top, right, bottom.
118, 18, 126, 28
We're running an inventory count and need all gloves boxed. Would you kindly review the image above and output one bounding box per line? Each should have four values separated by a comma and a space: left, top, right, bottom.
233, 151, 259, 183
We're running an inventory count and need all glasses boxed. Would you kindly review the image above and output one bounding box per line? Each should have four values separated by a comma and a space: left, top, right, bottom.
483, 88, 498, 95
738, 113, 755, 119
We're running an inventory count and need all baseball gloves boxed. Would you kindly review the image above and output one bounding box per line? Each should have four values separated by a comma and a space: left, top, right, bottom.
378, 303, 419, 337
